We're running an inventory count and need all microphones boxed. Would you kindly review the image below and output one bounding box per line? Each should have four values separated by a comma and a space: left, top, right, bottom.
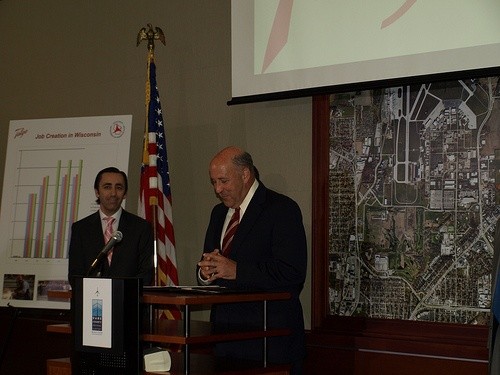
88, 231, 122, 274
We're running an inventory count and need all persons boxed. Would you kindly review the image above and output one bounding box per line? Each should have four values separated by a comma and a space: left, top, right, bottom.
11, 275, 33, 300
195, 145, 308, 375
68, 167, 156, 327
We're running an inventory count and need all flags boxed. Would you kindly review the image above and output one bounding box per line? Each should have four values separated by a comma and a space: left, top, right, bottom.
138, 53, 184, 321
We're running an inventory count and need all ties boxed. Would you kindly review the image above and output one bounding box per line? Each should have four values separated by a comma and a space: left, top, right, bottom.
104, 217, 116, 268
222, 208, 241, 258
